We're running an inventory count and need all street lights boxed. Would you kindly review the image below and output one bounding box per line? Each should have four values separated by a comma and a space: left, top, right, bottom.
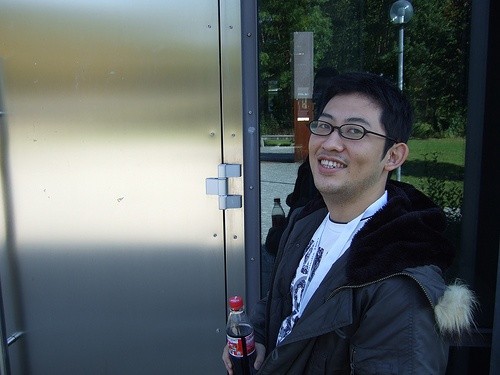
390, 1, 415, 182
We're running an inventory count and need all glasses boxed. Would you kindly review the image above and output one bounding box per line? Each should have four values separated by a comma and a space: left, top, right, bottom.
305, 119, 396, 143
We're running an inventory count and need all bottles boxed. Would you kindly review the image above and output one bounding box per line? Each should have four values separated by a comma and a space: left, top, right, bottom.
226, 296, 257, 375
272, 198, 286, 227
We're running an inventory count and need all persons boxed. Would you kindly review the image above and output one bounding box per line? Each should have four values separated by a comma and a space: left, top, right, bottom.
222, 73, 478, 375
311, 69, 339, 110
